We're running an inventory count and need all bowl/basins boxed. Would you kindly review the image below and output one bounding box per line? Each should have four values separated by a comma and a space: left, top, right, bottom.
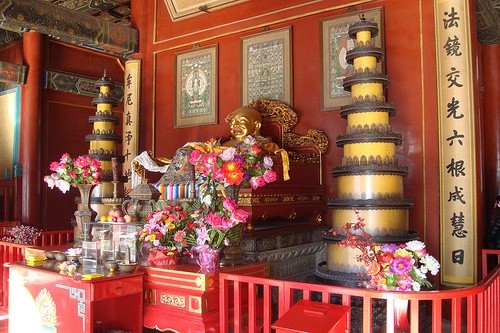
105, 262, 118, 271
65, 255, 81, 262
117, 262, 138, 273
53, 252, 70, 261
44, 250, 61, 259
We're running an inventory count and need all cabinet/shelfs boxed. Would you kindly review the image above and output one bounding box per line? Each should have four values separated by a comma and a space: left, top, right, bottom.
3, 259, 145, 333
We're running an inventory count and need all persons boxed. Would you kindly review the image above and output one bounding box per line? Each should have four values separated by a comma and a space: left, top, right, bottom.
191, 104, 274, 163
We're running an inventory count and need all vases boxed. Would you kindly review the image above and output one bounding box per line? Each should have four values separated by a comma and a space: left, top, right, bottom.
388, 286, 410, 333
196, 247, 224, 274
149, 245, 181, 266
220, 180, 243, 266
73, 181, 98, 248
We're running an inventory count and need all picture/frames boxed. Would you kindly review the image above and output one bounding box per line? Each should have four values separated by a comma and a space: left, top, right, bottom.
319, 6, 390, 112
239, 25, 294, 112
174, 43, 221, 128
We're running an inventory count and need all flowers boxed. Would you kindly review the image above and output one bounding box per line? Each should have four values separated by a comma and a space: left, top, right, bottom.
44, 152, 112, 201
328, 208, 441, 292
174, 192, 251, 249
189, 134, 276, 188
139, 206, 196, 254
1, 224, 41, 247
